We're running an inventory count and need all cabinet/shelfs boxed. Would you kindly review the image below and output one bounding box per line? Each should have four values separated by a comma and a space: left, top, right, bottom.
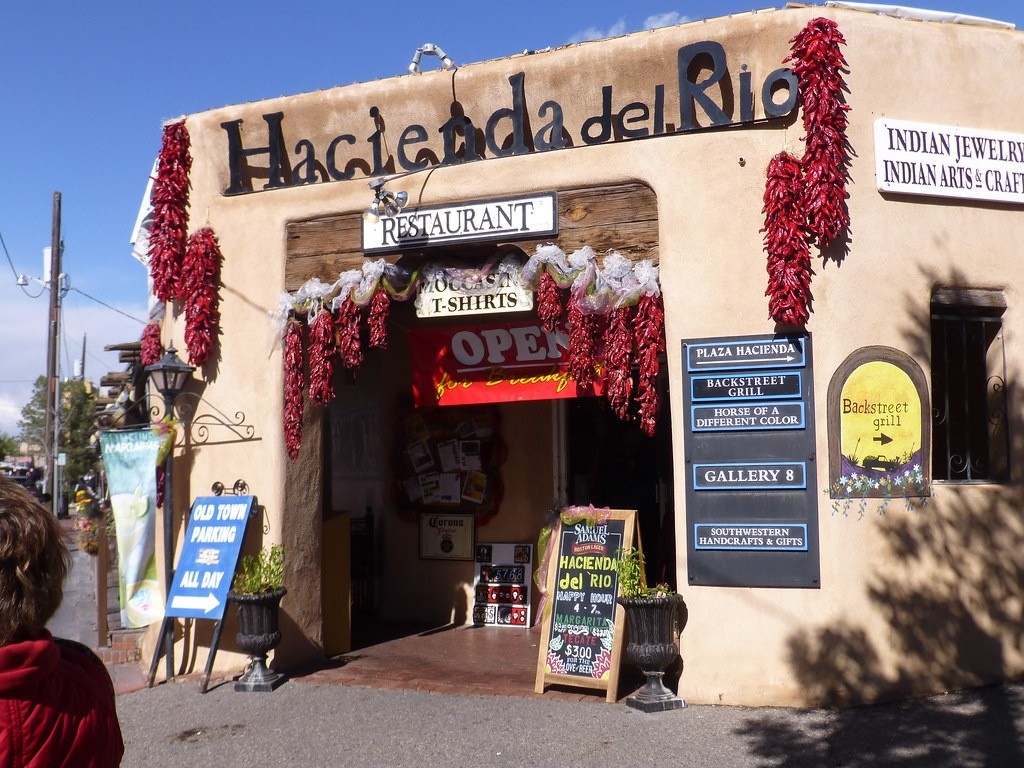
322, 511, 351, 652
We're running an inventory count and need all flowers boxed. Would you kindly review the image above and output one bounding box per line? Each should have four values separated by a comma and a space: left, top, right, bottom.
72, 490, 99, 533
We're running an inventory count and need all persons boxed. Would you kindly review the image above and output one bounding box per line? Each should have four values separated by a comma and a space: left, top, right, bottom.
0, 473, 124, 768
75, 470, 99, 500
25, 468, 38, 488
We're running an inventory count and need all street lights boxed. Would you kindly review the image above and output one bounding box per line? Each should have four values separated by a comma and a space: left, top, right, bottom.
142, 338, 197, 683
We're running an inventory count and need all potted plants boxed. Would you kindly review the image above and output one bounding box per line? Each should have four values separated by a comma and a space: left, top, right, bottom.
228, 544, 287, 692
610, 546, 687, 712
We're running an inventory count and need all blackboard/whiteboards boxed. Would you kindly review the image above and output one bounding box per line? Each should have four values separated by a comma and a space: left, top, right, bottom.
535, 510, 647, 690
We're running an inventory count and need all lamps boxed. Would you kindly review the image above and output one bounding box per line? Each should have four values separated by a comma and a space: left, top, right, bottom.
143, 339, 197, 401
363, 164, 441, 224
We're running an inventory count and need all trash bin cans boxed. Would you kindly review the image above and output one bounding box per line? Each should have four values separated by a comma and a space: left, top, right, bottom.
52, 492, 68, 519
